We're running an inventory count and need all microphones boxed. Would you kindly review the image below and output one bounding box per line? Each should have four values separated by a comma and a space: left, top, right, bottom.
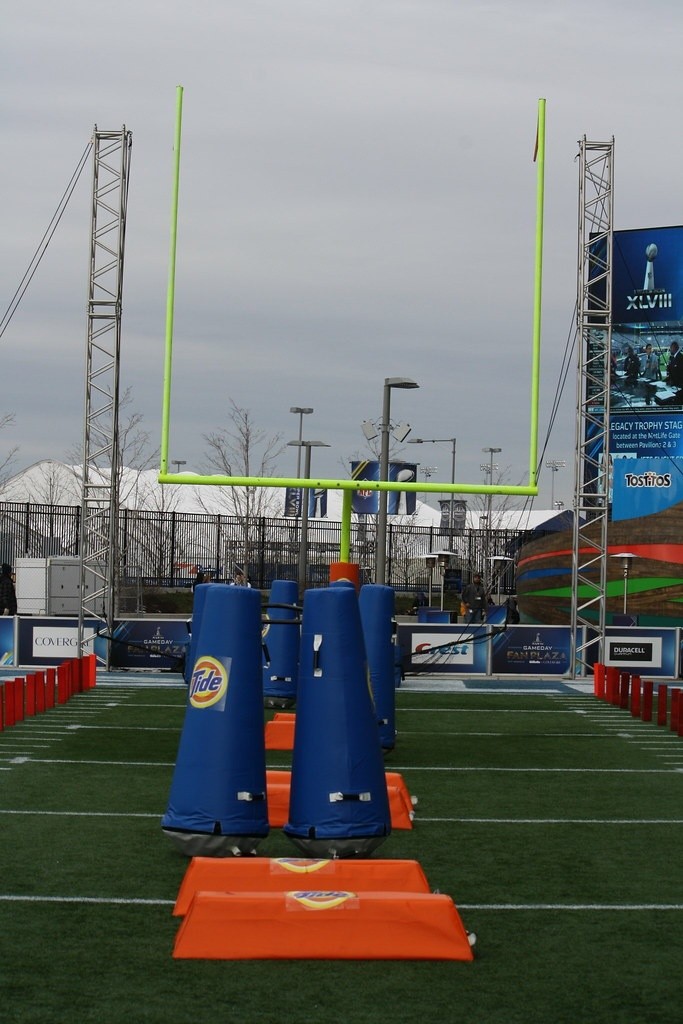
647, 355, 650, 363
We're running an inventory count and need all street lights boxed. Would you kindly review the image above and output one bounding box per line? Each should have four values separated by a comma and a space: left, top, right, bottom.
544, 459, 566, 509
285, 438, 331, 585
477, 444, 502, 529
405, 435, 458, 551
289, 406, 314, 541
552, 497, 565, 511
419, 466, 437, 506
479, 462, 501, 528
170, 458, 187, 474
372, 377, 418, 584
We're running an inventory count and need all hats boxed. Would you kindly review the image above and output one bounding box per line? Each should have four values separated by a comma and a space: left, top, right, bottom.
474, 573, 482, 576
2, 563, 12, 573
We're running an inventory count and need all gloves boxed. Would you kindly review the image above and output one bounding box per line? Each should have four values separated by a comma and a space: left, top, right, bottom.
3, 608, 9, 615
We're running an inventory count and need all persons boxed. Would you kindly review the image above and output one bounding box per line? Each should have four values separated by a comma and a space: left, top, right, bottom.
461, 574, 486, 624
623, 347, 641, 378
230, 573, 251, 588
639, 344, 658, 379
0, 563, 17, 616
662, 341, 683, 388
599, 453, 613, 499
193, 572, 211, 596
411, 592, 427, 615
